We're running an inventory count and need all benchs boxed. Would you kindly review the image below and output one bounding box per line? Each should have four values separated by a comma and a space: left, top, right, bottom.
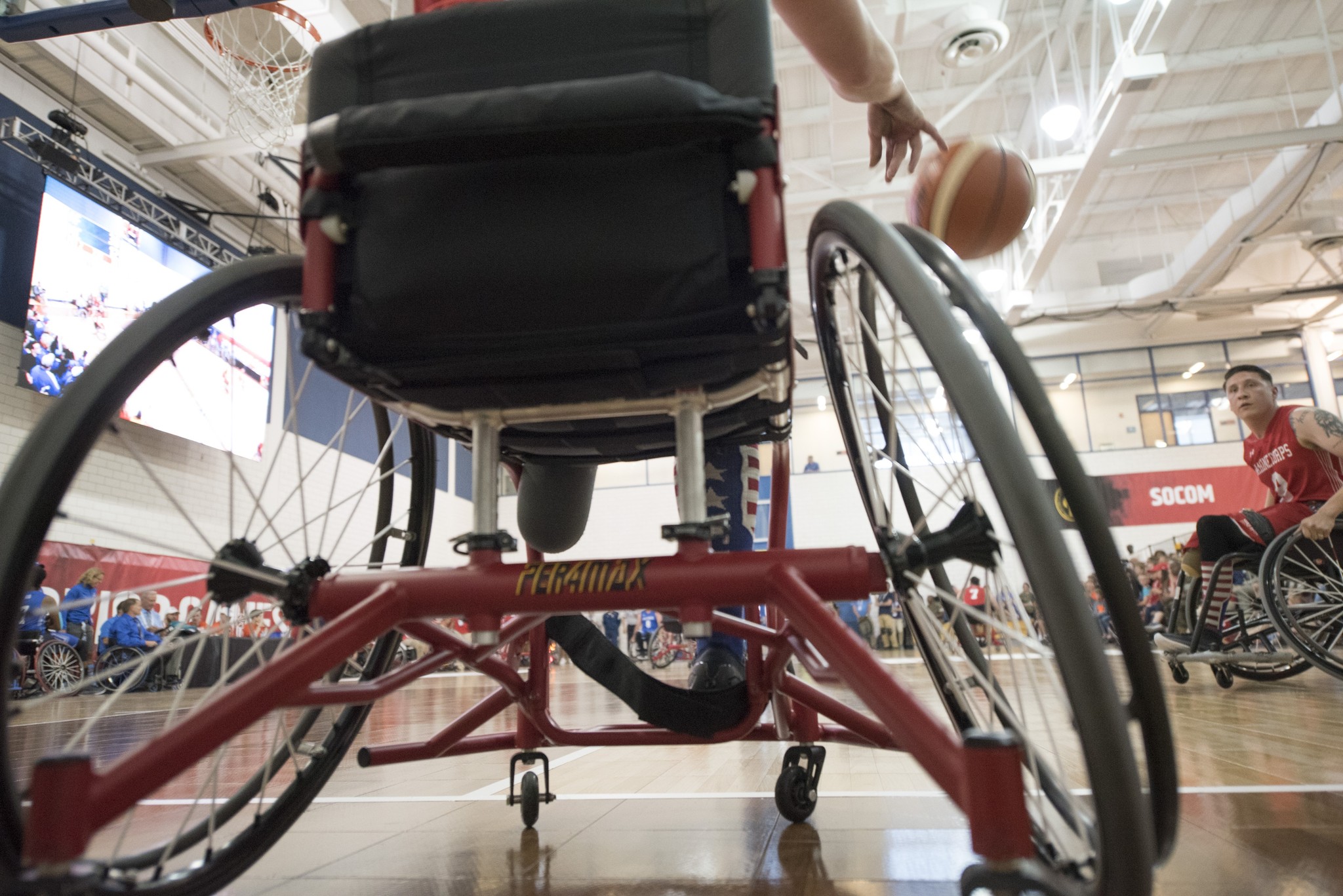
939, 615, 1033, 641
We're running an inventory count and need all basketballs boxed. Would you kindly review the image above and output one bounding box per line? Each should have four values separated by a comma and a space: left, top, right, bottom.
903, 132, 1039, 261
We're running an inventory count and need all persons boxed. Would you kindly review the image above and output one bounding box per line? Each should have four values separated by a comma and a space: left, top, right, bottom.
1153, 364, 1343, 656
597, 543, 1287, 653
13, 210, 277, 466
389, 1, 946, 734
7, 556, 565, 696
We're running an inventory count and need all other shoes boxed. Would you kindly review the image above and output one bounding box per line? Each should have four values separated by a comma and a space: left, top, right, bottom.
77, 682, 106, 696
166, 676, 183, 684
1143, 622, 1164, 634
687, 650, 743, 690
1153, 627, 1221, 656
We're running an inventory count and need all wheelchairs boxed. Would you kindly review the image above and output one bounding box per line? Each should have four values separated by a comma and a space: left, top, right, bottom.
1153, 519, 1343, 688
627, 622, 696, 670
92, 643, 166, 691
9, 630, 85, 698
343, 640, 407, 678
0, 2, 1180, 896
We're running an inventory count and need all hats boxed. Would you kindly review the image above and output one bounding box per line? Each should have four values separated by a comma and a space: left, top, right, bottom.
164, 606, 179, 616
1149, 563, 1168, 574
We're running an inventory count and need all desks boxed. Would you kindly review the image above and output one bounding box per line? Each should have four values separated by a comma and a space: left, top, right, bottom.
178, 636, 294, 686
